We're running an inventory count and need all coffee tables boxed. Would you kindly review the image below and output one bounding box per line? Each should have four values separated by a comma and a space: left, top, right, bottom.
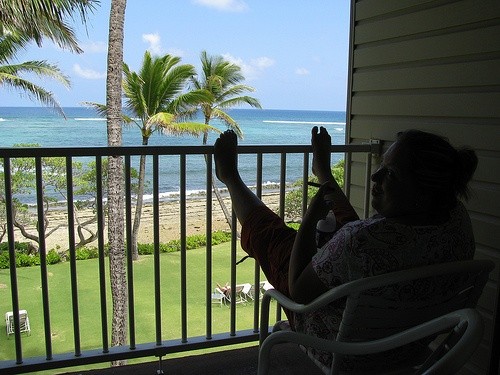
211, 294, 225, 307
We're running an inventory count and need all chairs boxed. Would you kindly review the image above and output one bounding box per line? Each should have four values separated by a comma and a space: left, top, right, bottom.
215, 281, 274, 306
257, 256, 496, 374
5, 310, 30, 338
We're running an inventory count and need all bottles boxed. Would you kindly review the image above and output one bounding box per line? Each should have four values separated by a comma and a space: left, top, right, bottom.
315, 205, 336, 252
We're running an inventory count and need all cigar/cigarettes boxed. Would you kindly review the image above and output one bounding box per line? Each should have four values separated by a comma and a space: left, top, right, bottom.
306, 182, 321, 187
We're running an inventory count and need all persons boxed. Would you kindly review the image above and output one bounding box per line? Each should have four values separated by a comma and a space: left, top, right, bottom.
216, 282, 231, 295
214, 126, 478, 375
